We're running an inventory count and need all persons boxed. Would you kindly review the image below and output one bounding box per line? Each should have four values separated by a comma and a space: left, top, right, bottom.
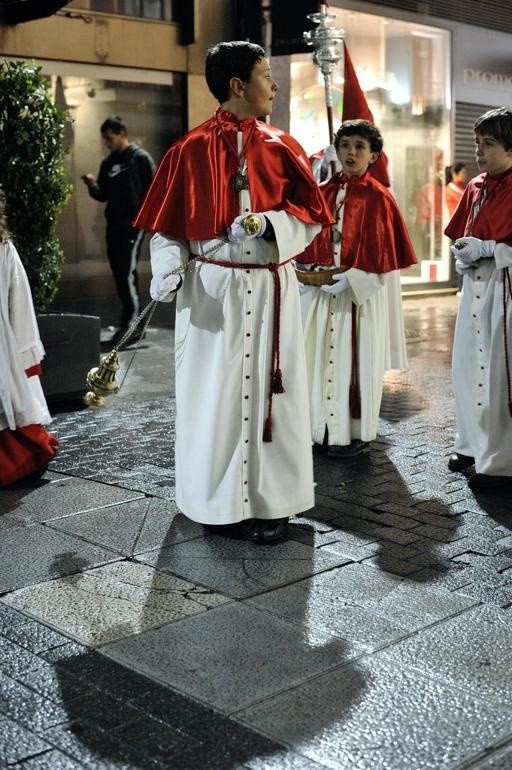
442, 106, 512, 491
127, 38, 340, 548
1, 228, 60, 486
298, 118, 420, 459
81, 114, 157, 347
308, 142, 346, 186
444, 161, 470, 223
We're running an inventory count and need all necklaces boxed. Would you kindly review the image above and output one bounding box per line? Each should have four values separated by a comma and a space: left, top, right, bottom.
475, 172, 501, 212
213, 113, 262, 192
327, 191, 354, 241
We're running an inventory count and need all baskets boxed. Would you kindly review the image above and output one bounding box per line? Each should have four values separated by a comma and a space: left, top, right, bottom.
296, 264, 348, 285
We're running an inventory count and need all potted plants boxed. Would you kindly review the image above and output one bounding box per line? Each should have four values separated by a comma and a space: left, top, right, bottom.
1, 52, 102, 405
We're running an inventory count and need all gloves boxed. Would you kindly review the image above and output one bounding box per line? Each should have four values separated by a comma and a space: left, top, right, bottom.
451, 237, 496, 262
149, 271, 181, 301
321, 274, 350, 294
321, 146, 337, 169
455, 260, 479, 275
227, 213, 266, 244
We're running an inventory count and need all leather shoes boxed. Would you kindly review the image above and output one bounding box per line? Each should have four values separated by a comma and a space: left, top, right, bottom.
329, 440, 372, 458
449, 453, 474, 471
248, 517, 288, 542
